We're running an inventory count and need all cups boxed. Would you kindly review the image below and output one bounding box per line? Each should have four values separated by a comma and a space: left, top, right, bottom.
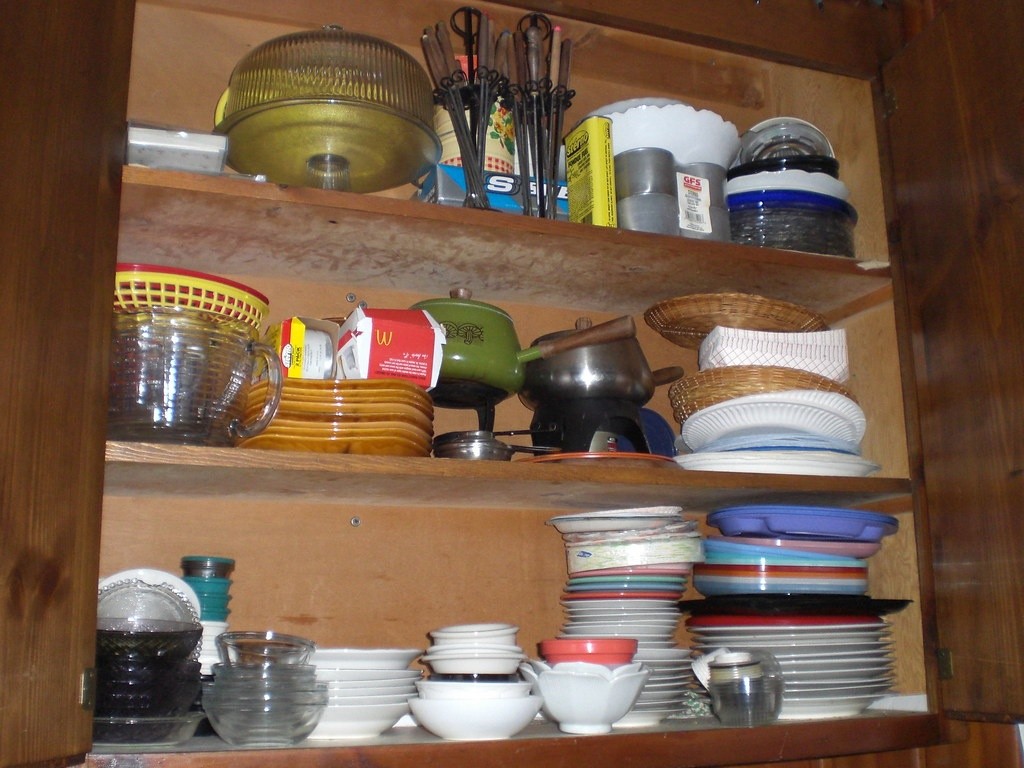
691, 647, 731, 690
617, 192, 680, 236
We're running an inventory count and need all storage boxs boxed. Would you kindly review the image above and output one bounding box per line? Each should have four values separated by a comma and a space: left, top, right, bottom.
409, 165, 569, 216
334, 303, 448, 393
251, 316, 340, 382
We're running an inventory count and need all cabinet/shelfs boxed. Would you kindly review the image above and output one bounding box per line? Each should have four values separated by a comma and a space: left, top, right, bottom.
0, 0, 1024, 768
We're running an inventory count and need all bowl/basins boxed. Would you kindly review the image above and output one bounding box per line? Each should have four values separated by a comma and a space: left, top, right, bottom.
677, 162, 726, 206
407, 622, 651, 741
615, 147, 677, 202
94, 618, 202, 741
303, 330, 333, 379
108, 305, 282, 449
180, 556, 329, 746
680, 206, 731, 244
604, 104, 743, 171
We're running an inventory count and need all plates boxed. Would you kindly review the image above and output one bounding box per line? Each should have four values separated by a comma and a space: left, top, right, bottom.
546, 504, 913, 728
96, 579, 202, 663
280, 645, 424, 742
232, 379, 435, 457
575, 97, 684, 127
674, 390, 883, 477
728, 117, 858, 259
97, 568, 201, 623
512, 451, 680, 467
92, 710, 205, 746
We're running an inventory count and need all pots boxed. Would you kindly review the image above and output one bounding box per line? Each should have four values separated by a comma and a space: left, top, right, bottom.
434, 425, 562, 462
518, 317, 684, 410
408, 287, 636, 400
